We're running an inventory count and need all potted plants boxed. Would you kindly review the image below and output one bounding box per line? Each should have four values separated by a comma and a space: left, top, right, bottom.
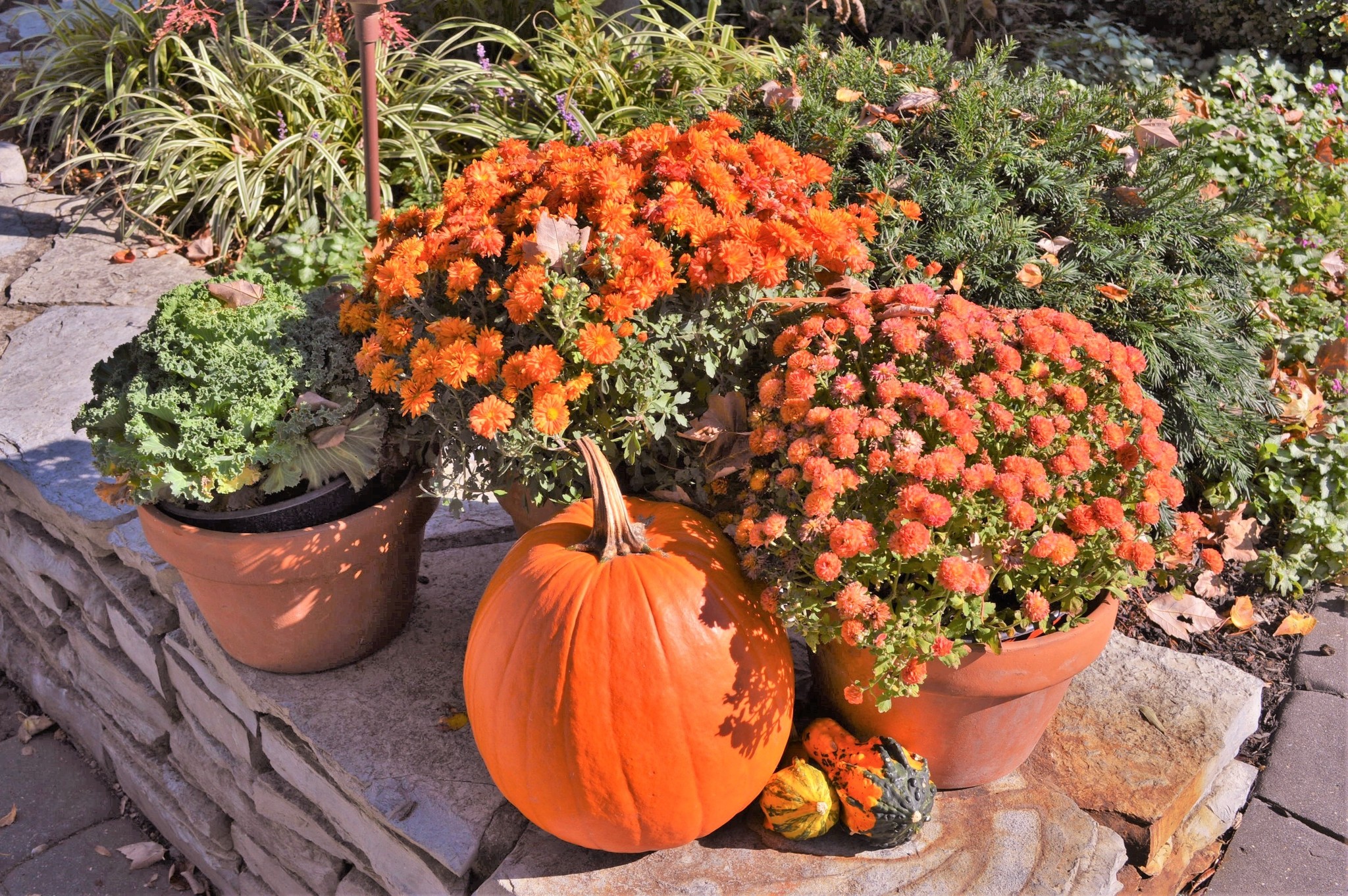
68, 268, 446, 676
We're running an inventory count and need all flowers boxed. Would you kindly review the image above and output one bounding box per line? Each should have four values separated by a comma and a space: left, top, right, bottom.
717, 284, 1222, 714
336, 107, 878, 523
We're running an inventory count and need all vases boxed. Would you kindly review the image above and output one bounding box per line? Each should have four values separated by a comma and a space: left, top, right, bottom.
479, 443, 585, 545
804, 591, 1121, 791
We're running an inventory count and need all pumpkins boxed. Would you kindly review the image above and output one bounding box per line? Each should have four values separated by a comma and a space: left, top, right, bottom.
462, 434, 937, 853
